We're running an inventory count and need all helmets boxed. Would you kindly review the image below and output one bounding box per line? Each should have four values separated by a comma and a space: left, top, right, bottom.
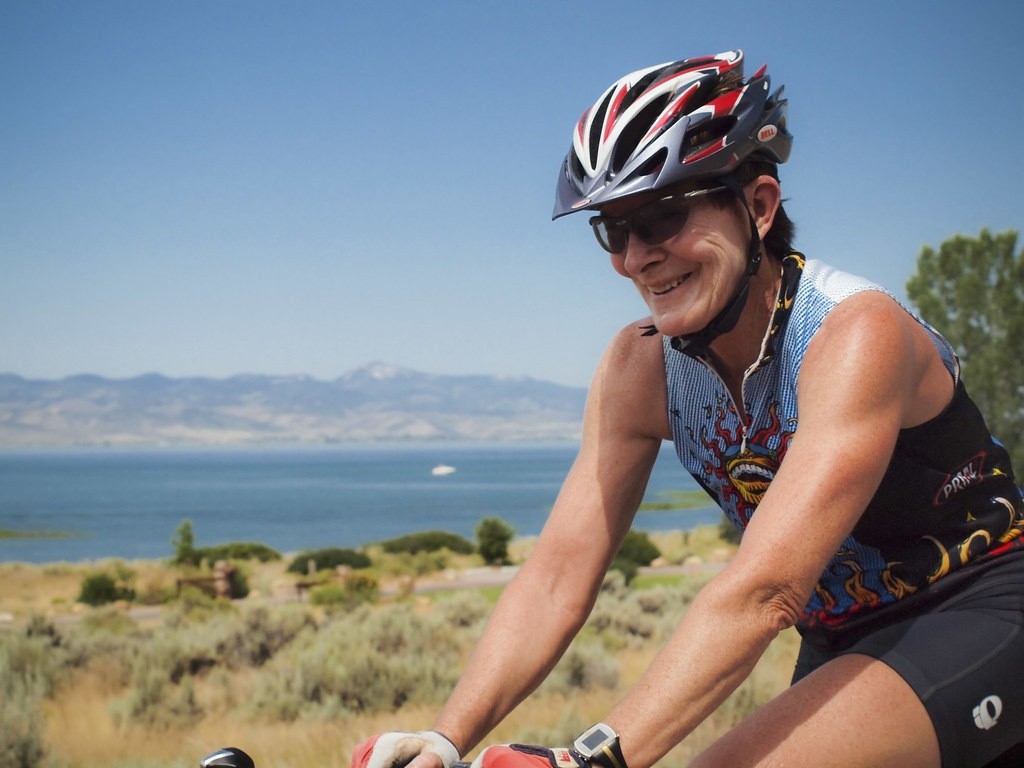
551, 49, 793, 219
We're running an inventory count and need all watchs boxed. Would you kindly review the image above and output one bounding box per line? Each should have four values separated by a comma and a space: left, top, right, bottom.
571, 723, 631, 768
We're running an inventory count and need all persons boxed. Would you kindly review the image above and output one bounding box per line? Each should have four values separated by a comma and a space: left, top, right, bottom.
348, 49, 1024, 768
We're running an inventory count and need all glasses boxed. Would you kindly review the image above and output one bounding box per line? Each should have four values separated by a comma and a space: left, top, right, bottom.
590, 181, 745, 256
